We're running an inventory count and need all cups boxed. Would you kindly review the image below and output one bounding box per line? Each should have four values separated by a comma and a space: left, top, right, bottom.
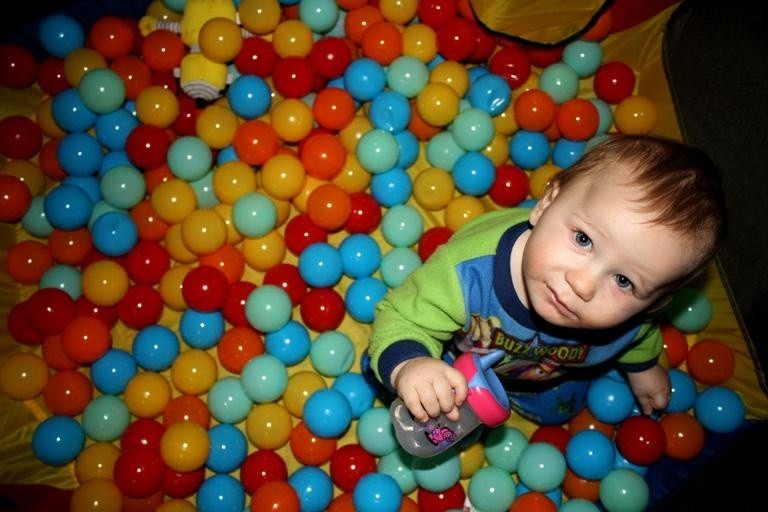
387, 348, 510, 460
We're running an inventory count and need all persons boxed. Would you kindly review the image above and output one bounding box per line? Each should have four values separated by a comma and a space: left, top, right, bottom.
369, 134, 727, 423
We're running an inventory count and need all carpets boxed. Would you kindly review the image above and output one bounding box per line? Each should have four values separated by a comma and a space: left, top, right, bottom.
661, 1, 767, 397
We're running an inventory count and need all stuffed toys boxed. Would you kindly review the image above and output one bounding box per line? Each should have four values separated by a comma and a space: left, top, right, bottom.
139, 0, 273, 108
